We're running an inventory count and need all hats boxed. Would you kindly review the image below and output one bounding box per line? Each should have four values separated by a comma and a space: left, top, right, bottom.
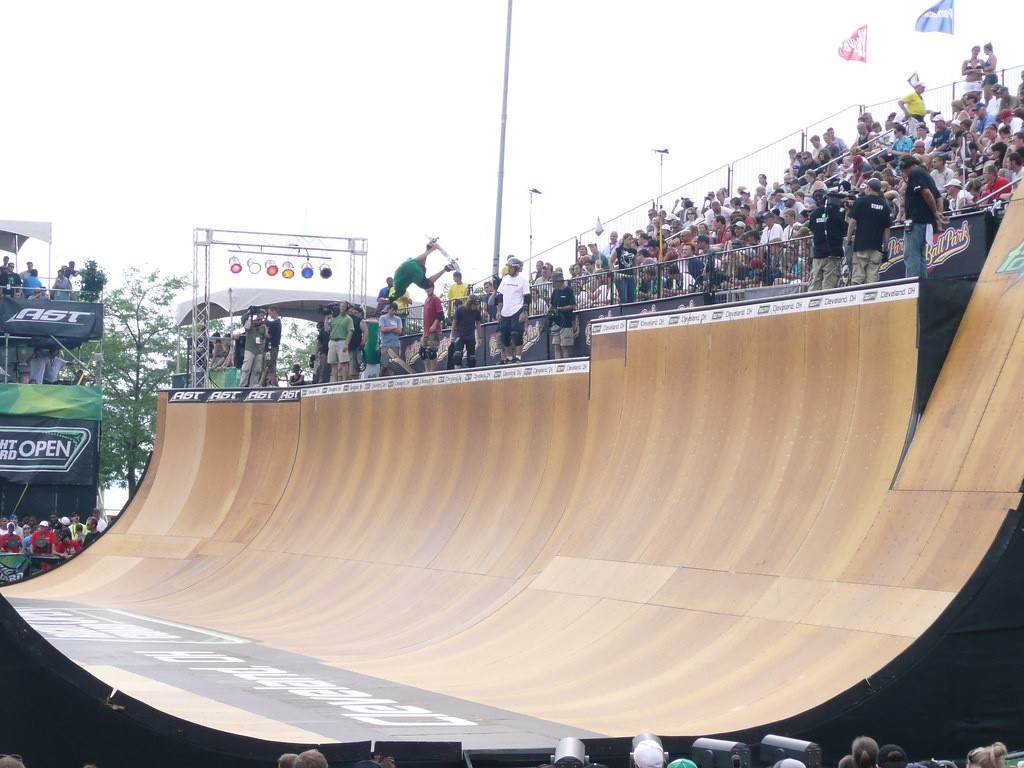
951, 120, 961, 127
693, 235, 709, 242
862, 177, 881, 193
576, 242, 598, 249
914, 81, 928, 88
853, 155, 863, 169
932, 115, 944, 122
680, 197, 692, 200
996, 87, 1008, 93
664, 214, 678, 221
39, 520, 50, 527
991, 83, 1002, 91
779, 193, 796, 202
57, 516, 71, 526
997, 109, 1016, 119
772, 758, 807, 768
943, 179, 963, 188
660, 224, 672, 232
9, 514, 18, 518
913, 140, 926, 147
761, 212, 774, 219
733, 220, 748, 230
633, 740, 664, 768
742, 189, 751, 195
667, 759, 698, 768
548, 272, 566, 283
916, 124, 929, 129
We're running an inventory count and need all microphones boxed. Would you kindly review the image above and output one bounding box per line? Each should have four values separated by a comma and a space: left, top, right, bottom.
848, 195, 856, 200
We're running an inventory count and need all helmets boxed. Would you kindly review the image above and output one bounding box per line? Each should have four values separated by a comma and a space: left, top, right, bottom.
505, 257, 525, 272
467, 294, 481, 305
256, 309, 268, 316
386, 301, 398, 308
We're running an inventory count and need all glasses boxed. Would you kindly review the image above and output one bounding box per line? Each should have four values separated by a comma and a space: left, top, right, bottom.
687, 213, 695, 215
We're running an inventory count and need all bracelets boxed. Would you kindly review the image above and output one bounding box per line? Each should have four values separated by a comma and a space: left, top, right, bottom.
523, 310, 528, 313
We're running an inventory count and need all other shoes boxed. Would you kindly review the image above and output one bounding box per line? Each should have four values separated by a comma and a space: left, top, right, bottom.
444, 263, 454, 274
427, 241, 433, 249
496, 357, 521, 364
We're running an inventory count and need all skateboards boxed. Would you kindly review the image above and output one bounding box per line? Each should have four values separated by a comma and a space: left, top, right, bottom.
424, 234, 461, 272
387, 349, 416, 376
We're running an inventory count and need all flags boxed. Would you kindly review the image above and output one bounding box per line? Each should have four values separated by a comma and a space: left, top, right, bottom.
912, 0, 952, 34
838, 25, 867, 61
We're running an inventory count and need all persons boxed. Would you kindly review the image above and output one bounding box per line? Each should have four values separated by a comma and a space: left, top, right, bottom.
0, 42, 1024, 768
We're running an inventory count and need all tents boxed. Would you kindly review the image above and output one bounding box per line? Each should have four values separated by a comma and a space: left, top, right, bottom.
177, 288, 424, 375
0, 219, 52, 299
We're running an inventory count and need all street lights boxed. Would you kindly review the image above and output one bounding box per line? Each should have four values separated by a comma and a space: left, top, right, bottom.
526, 188, 543, 286
652, 148, 670, 301
